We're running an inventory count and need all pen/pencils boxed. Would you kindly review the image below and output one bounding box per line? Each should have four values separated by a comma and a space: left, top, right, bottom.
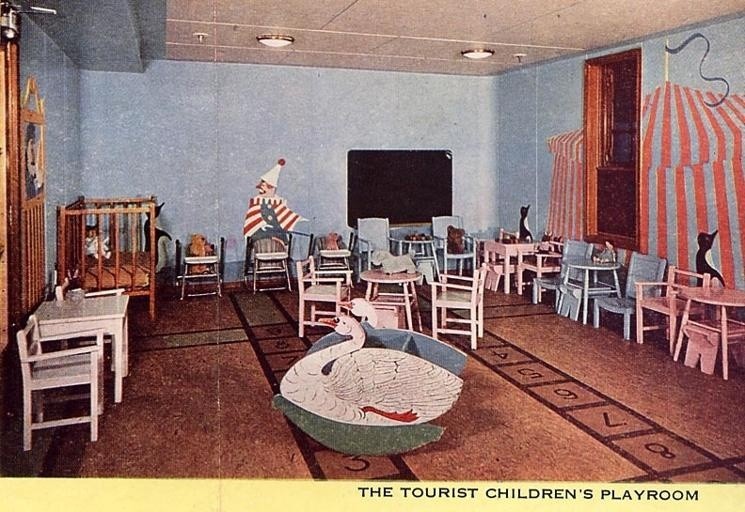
65, 267, 80, 282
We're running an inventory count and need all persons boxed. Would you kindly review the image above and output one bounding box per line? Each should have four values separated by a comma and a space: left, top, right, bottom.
189, 234, 215, 274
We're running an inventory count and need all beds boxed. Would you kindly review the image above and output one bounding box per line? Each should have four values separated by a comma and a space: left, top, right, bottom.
56, 192, 159, 323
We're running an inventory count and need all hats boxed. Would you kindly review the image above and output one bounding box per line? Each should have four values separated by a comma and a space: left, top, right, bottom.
261, 159, 284, 187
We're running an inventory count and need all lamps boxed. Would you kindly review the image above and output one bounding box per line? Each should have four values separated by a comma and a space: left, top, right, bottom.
257, 34, 295, 47
462, 48, 495, 60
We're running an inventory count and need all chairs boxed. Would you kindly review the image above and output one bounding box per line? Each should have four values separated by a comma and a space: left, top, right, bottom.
15, 312, 103, 454
55, 276, 127, 372
166, 216, 744, 379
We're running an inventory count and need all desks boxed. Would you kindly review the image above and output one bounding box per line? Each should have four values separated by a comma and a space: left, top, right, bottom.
34, 289, 132, 404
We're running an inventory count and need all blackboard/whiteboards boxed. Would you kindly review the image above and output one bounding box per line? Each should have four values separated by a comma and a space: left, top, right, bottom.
346, 148, 455, 231
